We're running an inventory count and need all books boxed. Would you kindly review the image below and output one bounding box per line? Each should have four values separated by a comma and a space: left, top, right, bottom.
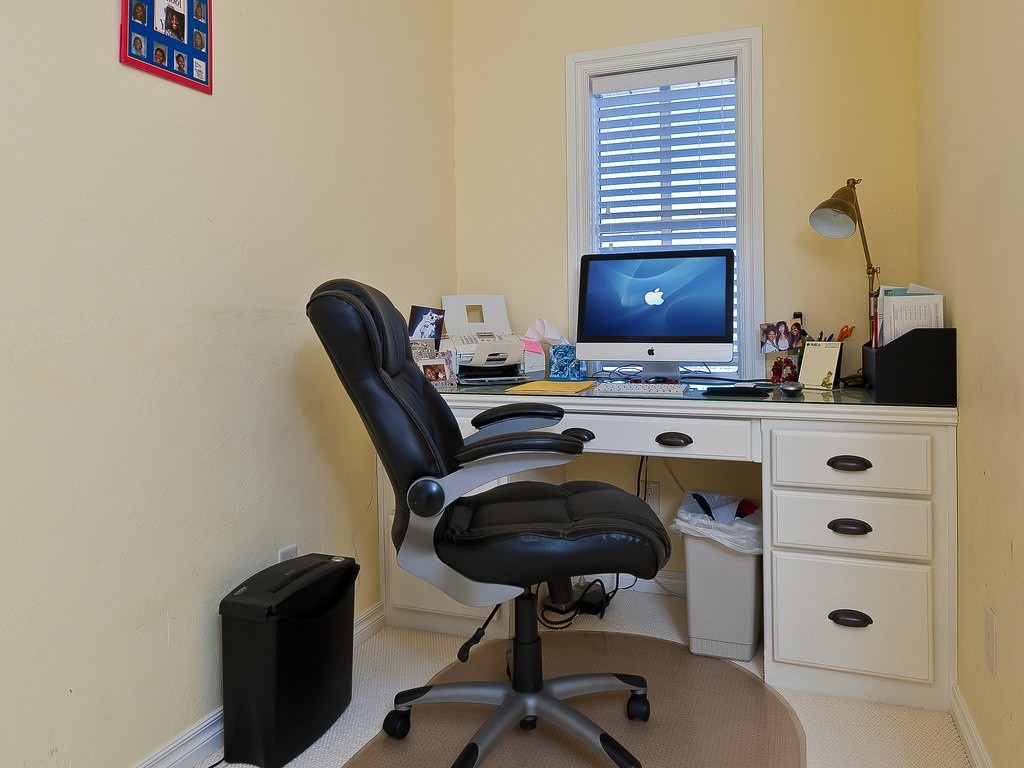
508, 377, 597, 395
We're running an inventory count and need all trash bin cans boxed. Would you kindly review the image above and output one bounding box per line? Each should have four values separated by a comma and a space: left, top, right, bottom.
676, 490, 763, 662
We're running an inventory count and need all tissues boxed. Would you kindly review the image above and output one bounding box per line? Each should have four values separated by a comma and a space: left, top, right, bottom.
522, 317, 588, 381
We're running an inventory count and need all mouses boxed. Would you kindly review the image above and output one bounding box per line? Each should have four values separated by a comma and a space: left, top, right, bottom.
780, 381, 804, 397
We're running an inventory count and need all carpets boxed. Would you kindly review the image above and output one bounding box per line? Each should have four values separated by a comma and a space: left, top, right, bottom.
344, 629, 809, 768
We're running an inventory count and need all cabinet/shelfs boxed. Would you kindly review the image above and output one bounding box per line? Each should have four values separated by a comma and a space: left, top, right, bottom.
378, 371, 957, 708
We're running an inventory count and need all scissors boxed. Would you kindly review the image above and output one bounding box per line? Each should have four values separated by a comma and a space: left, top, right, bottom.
837, 325, 856, 342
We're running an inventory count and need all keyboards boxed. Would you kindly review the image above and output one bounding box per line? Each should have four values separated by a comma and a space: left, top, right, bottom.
592, 383, 690, 396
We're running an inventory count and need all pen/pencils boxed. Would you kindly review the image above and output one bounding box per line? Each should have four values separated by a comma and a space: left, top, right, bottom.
799, 329, 833, 342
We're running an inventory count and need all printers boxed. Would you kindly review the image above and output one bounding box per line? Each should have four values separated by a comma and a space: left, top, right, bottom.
441, 294, 525, 367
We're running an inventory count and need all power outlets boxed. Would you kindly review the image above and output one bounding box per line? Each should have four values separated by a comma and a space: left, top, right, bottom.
640, 481, 659, 515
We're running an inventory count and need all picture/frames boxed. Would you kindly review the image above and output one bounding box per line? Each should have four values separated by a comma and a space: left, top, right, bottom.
118, 0, 213, 95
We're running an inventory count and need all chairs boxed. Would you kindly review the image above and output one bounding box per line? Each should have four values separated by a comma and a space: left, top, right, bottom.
307, 279, 671, 768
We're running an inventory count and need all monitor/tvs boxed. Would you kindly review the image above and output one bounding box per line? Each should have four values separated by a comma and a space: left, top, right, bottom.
577, 247, 734, 363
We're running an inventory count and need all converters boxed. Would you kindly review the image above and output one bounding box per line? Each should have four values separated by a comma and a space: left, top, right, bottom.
576, 590, 610, 616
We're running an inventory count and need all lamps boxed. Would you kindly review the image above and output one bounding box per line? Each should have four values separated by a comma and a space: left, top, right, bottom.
810, 178, 881, 387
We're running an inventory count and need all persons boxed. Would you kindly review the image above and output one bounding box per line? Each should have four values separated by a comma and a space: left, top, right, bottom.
760, 320, 805, 353
128, 0, 210, 79
425, 366, 446, 381
